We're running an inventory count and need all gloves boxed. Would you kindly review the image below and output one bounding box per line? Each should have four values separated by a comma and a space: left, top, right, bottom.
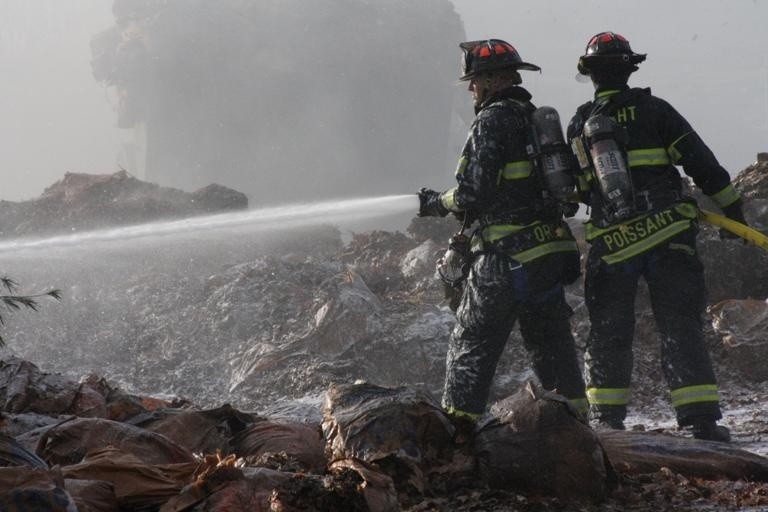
417, 187, 448, 217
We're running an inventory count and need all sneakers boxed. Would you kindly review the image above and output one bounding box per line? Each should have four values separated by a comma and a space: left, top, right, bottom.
695, 417, 730, 440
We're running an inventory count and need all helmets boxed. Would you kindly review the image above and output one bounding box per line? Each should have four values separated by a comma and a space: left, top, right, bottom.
459, 40, 540, 79
578, 32, 646, 73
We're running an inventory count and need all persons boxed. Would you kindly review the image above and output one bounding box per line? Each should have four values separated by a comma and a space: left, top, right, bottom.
417, 40, 592, 420
565, 30, 750, 445
